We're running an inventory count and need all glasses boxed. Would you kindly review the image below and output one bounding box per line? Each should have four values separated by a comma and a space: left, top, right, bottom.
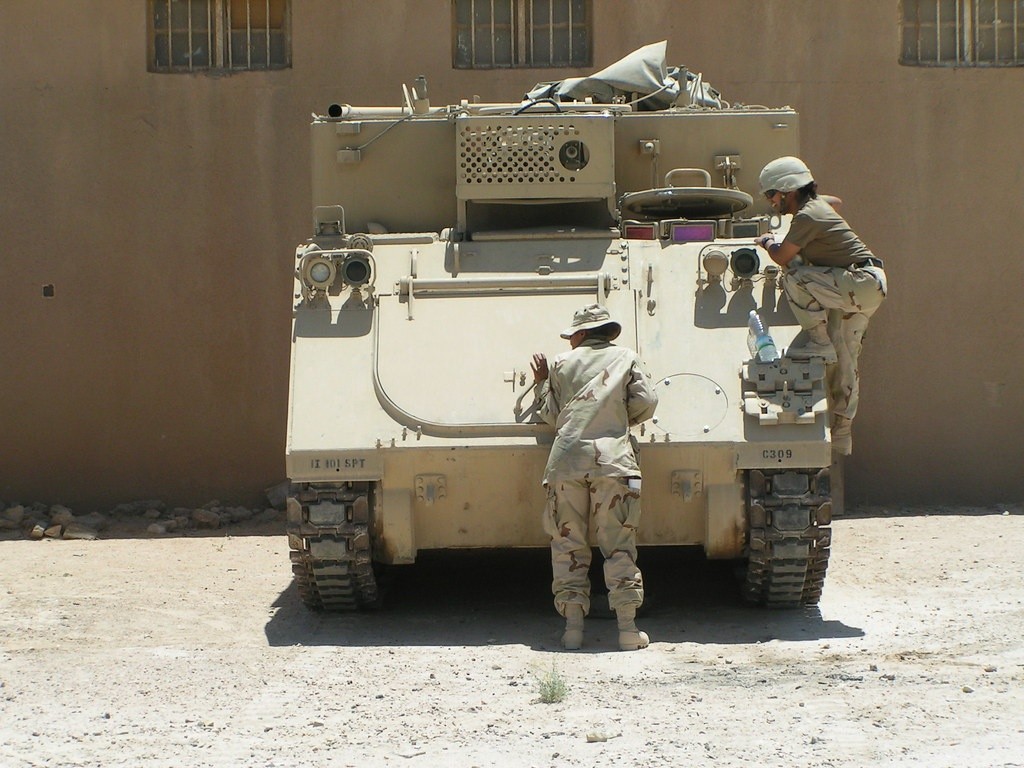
765, 189, 777, 199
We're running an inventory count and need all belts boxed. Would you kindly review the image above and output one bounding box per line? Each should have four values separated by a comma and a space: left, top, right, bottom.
857, 260, 882, 269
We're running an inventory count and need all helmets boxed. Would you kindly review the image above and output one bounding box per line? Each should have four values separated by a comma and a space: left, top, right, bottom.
759, 157, 814, 193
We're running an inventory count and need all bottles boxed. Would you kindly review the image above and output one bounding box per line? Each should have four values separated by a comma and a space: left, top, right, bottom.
748, 309, 779, 363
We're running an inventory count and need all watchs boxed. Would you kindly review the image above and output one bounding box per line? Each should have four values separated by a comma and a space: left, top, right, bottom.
761, 237, 773, 250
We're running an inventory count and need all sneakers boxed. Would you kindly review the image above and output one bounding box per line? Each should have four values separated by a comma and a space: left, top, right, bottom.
829, 436, 852, 455
784, 341, 837, 364
619, 627, 649, 651
561, 629, 583, 649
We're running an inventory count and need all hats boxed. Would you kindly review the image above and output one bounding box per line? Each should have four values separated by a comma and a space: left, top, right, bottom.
560, 302, 622, 341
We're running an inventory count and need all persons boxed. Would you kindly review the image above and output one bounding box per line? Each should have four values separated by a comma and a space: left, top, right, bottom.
533, 305, 657, 650
753, 156, 886, 455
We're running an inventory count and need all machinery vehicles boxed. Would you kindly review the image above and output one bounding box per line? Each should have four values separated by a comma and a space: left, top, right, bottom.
284, 42, 836, 616
